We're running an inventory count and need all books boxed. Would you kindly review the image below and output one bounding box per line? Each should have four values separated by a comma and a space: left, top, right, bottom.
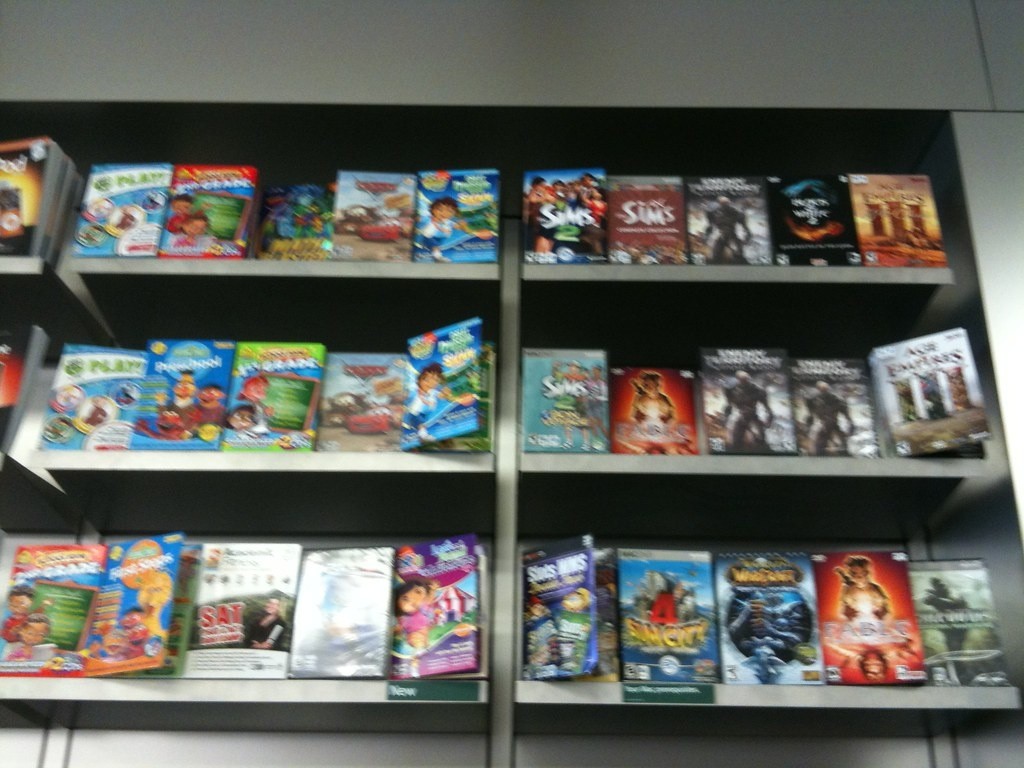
1, 136, 1008, 684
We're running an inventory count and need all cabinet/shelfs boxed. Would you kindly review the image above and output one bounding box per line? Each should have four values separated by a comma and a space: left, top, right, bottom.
0, 0, 1024, 768
